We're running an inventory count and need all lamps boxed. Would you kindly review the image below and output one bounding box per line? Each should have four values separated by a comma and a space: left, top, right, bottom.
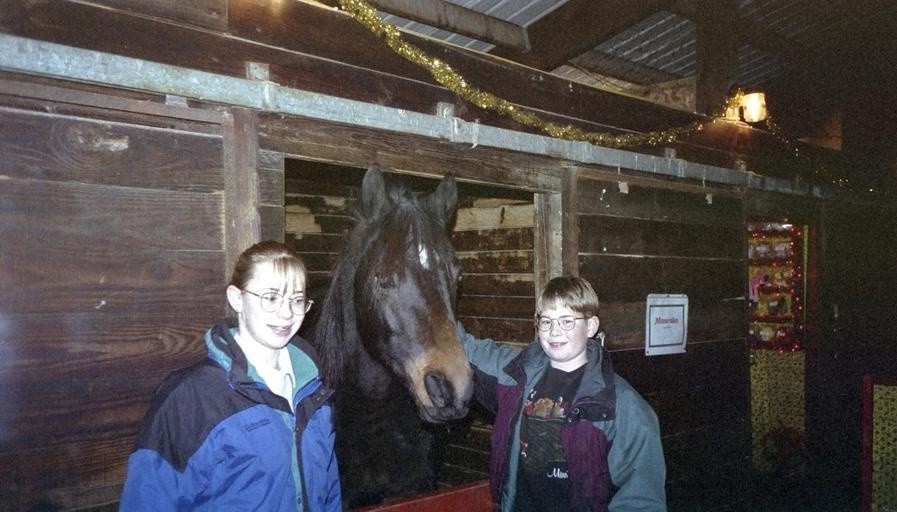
729, 85, 766, 123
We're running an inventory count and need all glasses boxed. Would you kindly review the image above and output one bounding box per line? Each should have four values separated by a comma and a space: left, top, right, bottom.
242, 288, 314, 315
537, 315, 591, 331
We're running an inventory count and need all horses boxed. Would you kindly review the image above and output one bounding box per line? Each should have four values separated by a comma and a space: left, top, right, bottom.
312, 161, 478, 511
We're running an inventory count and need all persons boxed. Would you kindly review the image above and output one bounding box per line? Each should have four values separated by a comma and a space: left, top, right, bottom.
456, 274, 671, 512
116, 237, 343, 512
